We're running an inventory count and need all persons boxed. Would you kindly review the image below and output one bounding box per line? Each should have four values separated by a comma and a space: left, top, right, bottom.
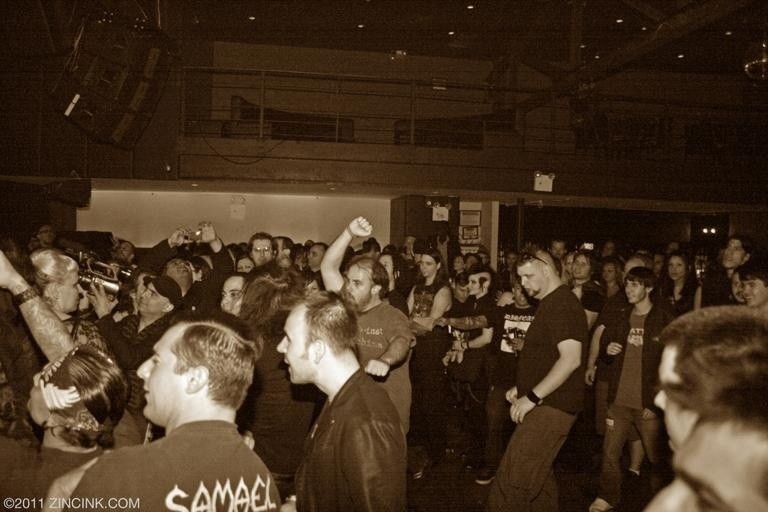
0, 216, 768, 512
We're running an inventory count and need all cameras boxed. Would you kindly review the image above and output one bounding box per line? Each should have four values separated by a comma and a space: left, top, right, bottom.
187, 231, 202, 240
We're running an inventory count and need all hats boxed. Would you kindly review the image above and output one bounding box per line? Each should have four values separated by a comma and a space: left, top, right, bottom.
144, 276, 181, 304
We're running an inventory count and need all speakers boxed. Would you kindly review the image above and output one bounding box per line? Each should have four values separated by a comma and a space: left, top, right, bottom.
391, 196, 460, 249
53, 30, 175, 150
